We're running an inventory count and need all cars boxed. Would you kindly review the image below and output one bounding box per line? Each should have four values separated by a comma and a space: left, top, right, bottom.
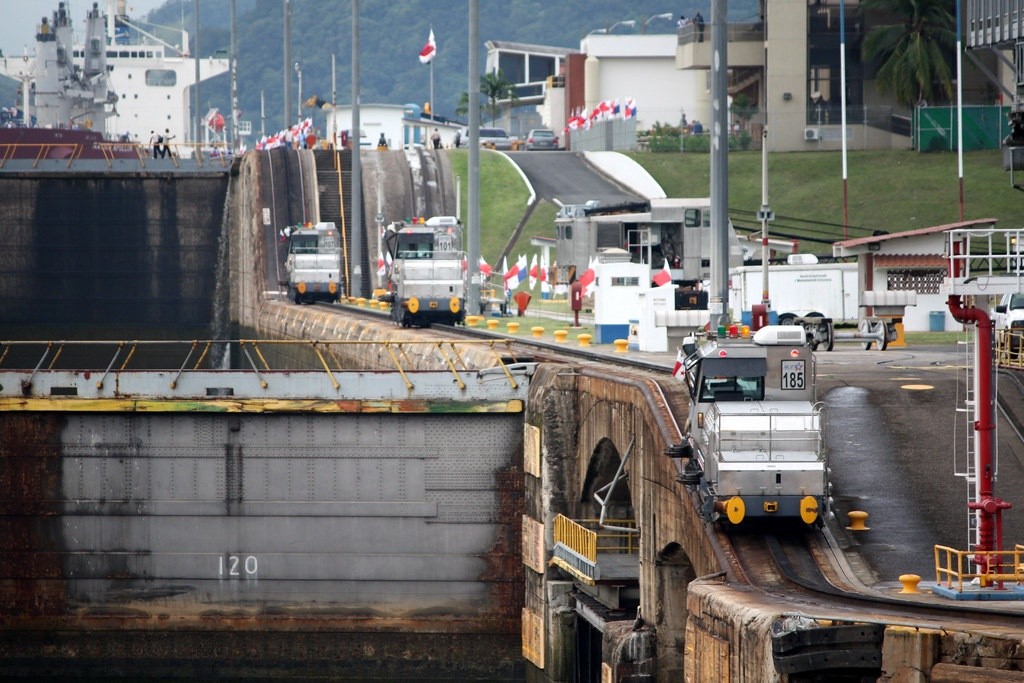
990, 292, 1024, 359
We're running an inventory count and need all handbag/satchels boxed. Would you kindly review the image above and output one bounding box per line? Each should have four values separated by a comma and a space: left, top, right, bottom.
157, 134, 164, 143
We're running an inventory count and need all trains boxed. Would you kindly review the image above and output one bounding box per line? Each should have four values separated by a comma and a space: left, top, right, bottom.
285, 222, 344, 305
663, 325, 835, 524
384, 216, 467, 328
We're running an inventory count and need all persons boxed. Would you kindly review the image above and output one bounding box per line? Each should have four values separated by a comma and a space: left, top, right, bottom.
733, 121, 741, 130
692, 11, 705, 42
749, 15, 764, 31
430, 127, 441, 149
677, 16, 689, 29
116, 128, 177, 160
689, 119, 702, 133
453, 129, 462, 148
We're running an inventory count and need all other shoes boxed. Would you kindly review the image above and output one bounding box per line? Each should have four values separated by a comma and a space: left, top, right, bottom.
151, 157, 158, 160
163, 157, 166, 160
169, 157, 173, 160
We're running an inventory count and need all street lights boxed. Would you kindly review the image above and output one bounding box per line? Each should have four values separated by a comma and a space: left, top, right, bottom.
587, 13, 673, 34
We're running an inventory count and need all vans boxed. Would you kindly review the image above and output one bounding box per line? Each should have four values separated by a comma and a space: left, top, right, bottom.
526, 129, 558, 151
460, 126, 518, 151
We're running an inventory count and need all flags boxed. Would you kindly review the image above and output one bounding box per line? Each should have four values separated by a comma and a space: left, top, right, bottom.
418, 22, 437, 64
672, 346, 688, 381
376, 250, 394, 278
652, 258, 671, 287
462, 253, 599, 298
240, 116, 314, 160
561, 97, 621, 135
624, 96, 637, 120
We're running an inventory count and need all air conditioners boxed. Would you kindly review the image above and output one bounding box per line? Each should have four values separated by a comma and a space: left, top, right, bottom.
803, 127, 819, 140
